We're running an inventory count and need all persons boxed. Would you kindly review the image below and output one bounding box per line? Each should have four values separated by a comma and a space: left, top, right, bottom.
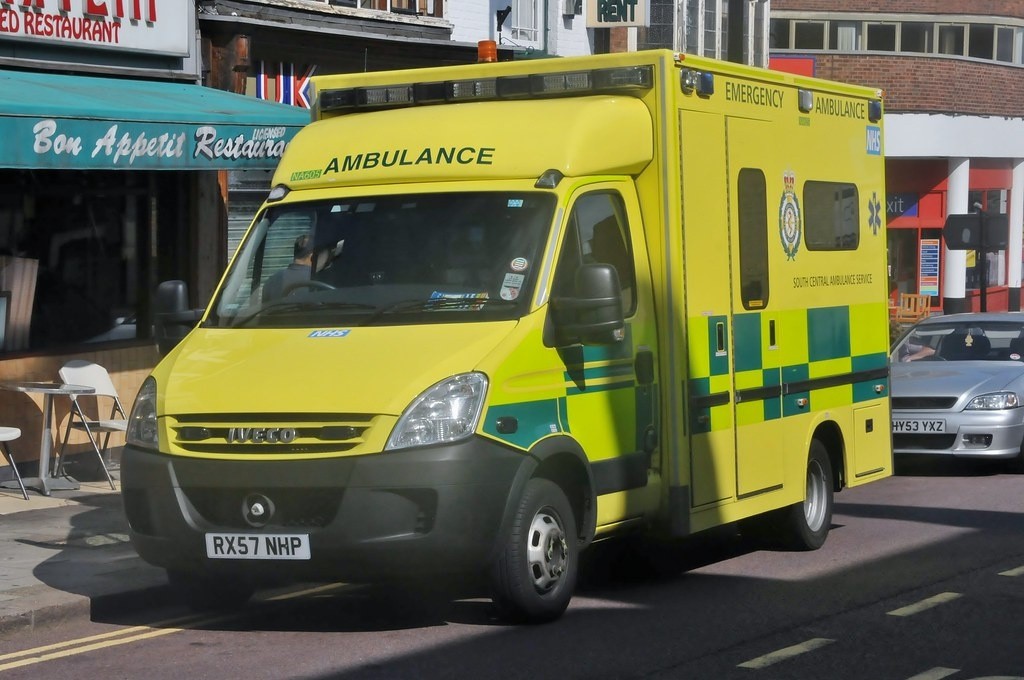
261, 233, 315, 303
889, 319, 936, 363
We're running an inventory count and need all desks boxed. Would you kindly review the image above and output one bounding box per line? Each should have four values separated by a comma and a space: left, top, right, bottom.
0, 383, 95, 495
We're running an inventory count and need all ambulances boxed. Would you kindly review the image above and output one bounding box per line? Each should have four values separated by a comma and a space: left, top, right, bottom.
116, 39, 892, 624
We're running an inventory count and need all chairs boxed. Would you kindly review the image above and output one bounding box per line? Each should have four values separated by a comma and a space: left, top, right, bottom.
940, 328, 992, 362
1, 426, 30, 500
55, 359, 129, 491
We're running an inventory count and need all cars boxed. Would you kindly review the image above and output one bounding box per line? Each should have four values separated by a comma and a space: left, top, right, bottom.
890, 312, 1024, 472
